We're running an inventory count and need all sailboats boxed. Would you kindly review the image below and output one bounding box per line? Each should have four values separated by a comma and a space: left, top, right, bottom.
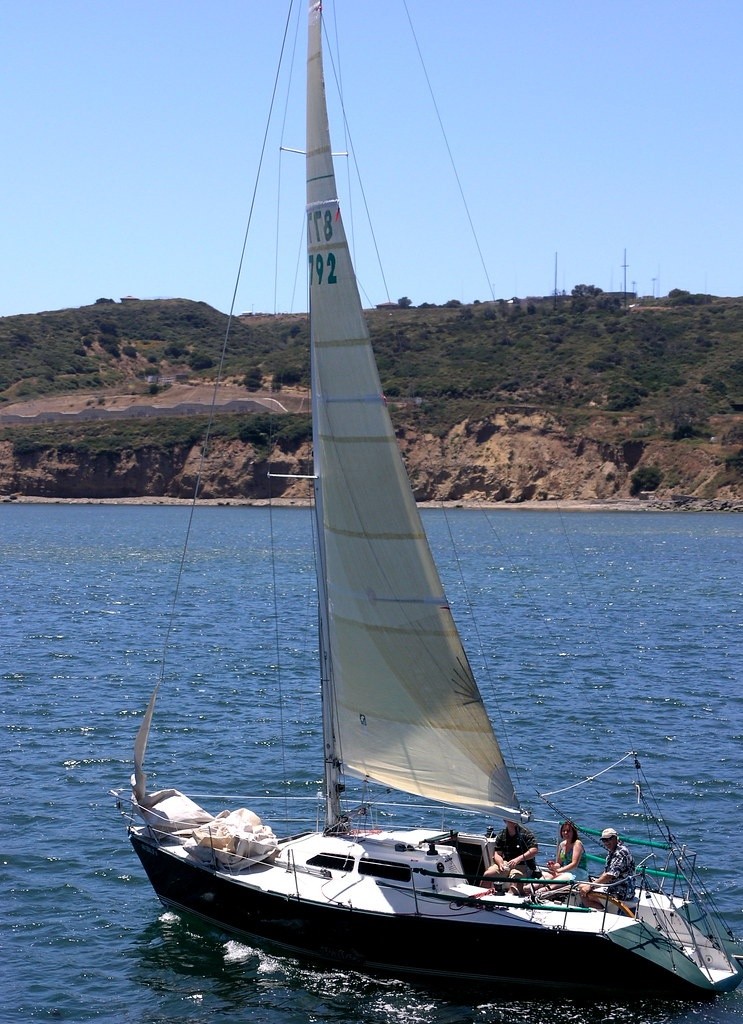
104, 0, 743, 1010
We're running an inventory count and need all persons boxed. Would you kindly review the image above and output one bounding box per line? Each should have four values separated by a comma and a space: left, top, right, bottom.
479, 815, 538, 894
511, 820, 587, 895
578, 827, 635, 912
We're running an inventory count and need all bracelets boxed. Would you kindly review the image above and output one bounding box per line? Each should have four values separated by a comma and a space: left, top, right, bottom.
590, 885, 595, 889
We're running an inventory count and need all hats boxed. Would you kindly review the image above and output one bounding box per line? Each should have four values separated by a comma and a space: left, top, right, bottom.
599, 828, 618, 841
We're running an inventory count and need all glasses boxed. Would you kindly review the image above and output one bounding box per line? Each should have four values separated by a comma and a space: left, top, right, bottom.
562, 828, 573, 833
602, 837, 615, 842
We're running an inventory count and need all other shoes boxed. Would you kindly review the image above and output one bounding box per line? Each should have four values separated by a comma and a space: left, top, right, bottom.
511, 885, 519, 895
